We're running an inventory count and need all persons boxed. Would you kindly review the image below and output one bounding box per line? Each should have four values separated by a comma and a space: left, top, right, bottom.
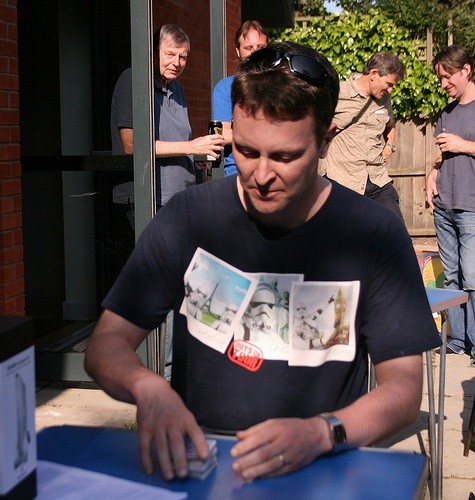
110, 21, 224, 232
211, 21, 270, 176
422, 47, 475, 364
83, 41, 444, 481
324, 52, 407, 219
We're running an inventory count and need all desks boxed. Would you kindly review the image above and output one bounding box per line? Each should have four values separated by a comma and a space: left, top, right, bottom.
368, 284, 470, 500
37, 424, 432, 500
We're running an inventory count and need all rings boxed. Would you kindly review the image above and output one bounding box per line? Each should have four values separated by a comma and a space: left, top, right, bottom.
278, 455, 286, 467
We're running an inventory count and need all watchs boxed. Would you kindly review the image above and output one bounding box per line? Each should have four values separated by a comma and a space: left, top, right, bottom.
320, 410, 349, 455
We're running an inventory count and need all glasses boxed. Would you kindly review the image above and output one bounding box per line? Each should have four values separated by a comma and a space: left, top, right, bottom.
243, 47, 335, 89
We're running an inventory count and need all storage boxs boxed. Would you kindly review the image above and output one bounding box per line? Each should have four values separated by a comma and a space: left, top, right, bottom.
0, 312, 37, 500
